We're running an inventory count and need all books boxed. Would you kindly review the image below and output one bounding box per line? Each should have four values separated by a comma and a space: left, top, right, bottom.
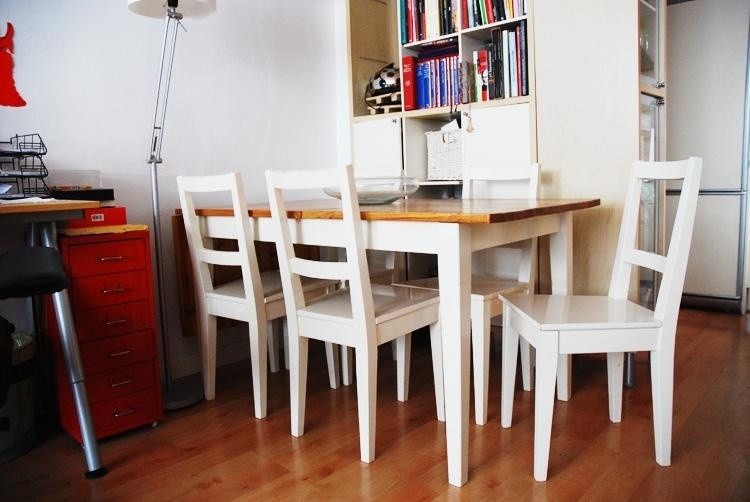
400, 0, 530, 111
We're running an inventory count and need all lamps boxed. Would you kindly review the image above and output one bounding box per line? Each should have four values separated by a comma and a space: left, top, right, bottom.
127, 1, 216, 424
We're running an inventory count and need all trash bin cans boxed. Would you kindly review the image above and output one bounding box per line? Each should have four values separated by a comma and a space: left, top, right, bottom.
0, 356, 38, 462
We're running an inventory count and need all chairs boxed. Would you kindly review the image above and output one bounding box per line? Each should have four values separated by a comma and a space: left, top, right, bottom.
175, 170, 347, 421
261, 162, 449, 464
331, 175, 412, 405
390, 160, 543, 429
0, 245, 106, 480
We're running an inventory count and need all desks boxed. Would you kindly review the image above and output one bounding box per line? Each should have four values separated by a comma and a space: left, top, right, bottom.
173, 188, 605, 487
0, 198, 102, 479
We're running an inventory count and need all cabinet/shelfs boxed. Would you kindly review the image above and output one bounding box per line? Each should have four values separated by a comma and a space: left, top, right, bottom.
44, 222, 163, 446
0, 132, 55, 197
347, 0, 540, 204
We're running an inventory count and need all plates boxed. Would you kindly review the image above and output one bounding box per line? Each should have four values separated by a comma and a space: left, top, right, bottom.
322, 179, 421, 205
